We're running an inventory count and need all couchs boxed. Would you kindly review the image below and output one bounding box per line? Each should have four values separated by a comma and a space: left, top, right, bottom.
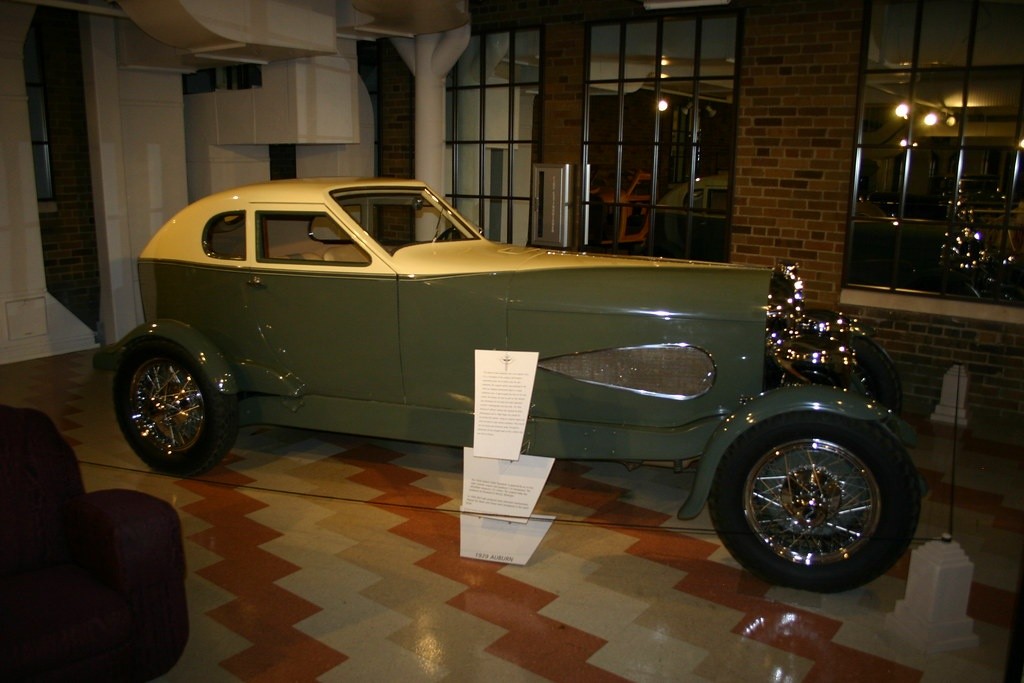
0, 403, 190, 683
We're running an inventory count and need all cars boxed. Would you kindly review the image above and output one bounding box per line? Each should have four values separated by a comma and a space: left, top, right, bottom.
650, 178, 727, 260
92, 175, 924, 595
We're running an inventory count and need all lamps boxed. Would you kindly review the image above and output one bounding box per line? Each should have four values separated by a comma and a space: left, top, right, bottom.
705, 106, 717, 118
681, 101, 693, 115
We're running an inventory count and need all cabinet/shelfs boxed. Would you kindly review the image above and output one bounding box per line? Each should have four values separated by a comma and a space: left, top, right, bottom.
216, 39, 361, 145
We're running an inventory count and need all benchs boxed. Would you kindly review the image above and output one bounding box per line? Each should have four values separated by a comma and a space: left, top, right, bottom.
269, 239, 327, 258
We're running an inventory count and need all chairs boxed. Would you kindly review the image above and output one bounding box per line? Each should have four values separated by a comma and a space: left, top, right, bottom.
276, 251, 322, 260
323, 245, 392, 263
